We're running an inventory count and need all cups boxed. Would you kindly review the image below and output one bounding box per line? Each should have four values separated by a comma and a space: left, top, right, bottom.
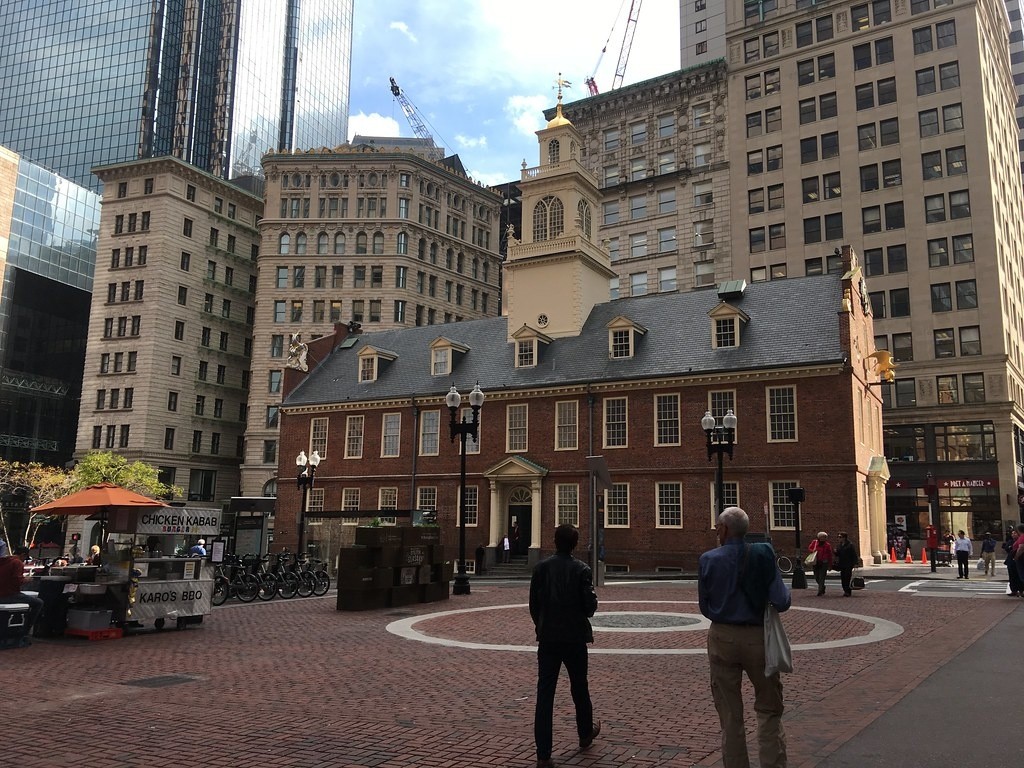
152, 550, 163, 558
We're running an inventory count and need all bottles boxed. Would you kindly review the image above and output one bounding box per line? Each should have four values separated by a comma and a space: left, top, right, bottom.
144, 546, 149, 557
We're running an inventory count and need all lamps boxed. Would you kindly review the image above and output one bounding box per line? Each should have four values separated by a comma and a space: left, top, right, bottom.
864, 349, 900, 386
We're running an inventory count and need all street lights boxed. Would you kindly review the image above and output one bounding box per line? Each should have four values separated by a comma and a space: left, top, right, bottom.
702, 410, 738, 521
294, 449, 321, 561
446, 379, 484, 595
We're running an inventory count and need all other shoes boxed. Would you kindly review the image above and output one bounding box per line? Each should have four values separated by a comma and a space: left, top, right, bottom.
1016, 590, 1024, 596
1007, 591, 1016, 596
817, 586, 826, 596
843, 592, 850, 597
537, 757, 553, 768
957, 575, 964, 579
579, 719, 601, 748
965, 576, 970, 579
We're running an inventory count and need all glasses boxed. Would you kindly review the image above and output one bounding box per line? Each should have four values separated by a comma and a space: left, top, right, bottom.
837, 536, 845, 539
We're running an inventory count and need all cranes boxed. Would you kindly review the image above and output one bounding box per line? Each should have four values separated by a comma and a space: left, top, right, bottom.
582, 0, 642, 99
389, 76, 477, 183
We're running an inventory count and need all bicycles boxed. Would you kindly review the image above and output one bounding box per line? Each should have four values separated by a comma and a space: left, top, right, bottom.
774, 549, 793, 573
212, 552, 330, 606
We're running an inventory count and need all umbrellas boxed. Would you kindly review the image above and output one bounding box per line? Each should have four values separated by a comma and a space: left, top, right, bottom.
29, 478, 171, 565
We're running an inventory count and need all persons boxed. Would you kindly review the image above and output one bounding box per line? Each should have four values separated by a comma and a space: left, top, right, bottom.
475, 544, 485, 575
831, 532, 861, 596
512, 522, 521, 555
0, 528, 44, 647
497, 534, 512, 563
888, 529, 910, 559
954, 530, 972, 579
1004, 525, 1024, 597
529, 524, 601, 768
808, 532, 832, 596
980, 533, 997, 576
699, 506, 791, 768
942, 531, 954, 565
189, 539, 206, 557
72, 545, 100, 565
145, 536, 159, 551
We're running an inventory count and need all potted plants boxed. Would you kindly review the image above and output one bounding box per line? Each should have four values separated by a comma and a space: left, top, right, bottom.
355, 515, 403, 548
400, 520, 442, 546
430, 559, 454, 583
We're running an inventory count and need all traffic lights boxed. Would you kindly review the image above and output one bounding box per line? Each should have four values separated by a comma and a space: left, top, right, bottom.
71, 533, 81, 540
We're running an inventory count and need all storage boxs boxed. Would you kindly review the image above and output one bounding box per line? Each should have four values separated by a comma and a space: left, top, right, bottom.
38, 576, 72, 603
79, 584, 107, 594
0, 603, 33, 640
62, 565, 97, 582
67, 606, 113, 630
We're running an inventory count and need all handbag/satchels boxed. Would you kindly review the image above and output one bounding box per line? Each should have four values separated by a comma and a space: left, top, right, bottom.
977, 557, 986, 570
851, 577, 865, 590
805, 540, 818, 567
763, 600, 794, 677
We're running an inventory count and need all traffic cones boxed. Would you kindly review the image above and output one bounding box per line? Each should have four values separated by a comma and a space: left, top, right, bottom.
904, 547, 914, 564
890, 546, 898, 563
920, 548, 928, 564
